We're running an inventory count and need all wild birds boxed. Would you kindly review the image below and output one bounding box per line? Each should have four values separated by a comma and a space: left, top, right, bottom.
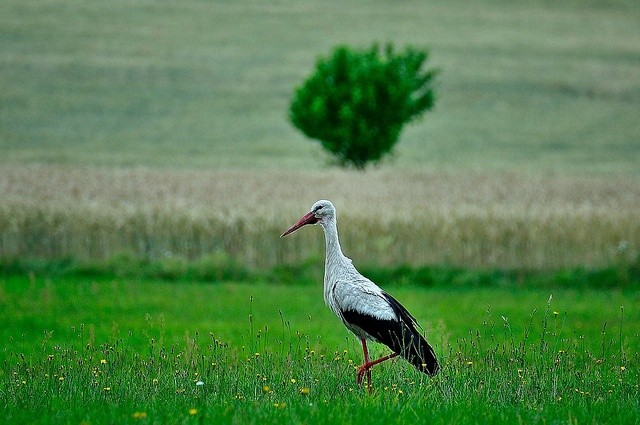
280, 200, 440, 395
